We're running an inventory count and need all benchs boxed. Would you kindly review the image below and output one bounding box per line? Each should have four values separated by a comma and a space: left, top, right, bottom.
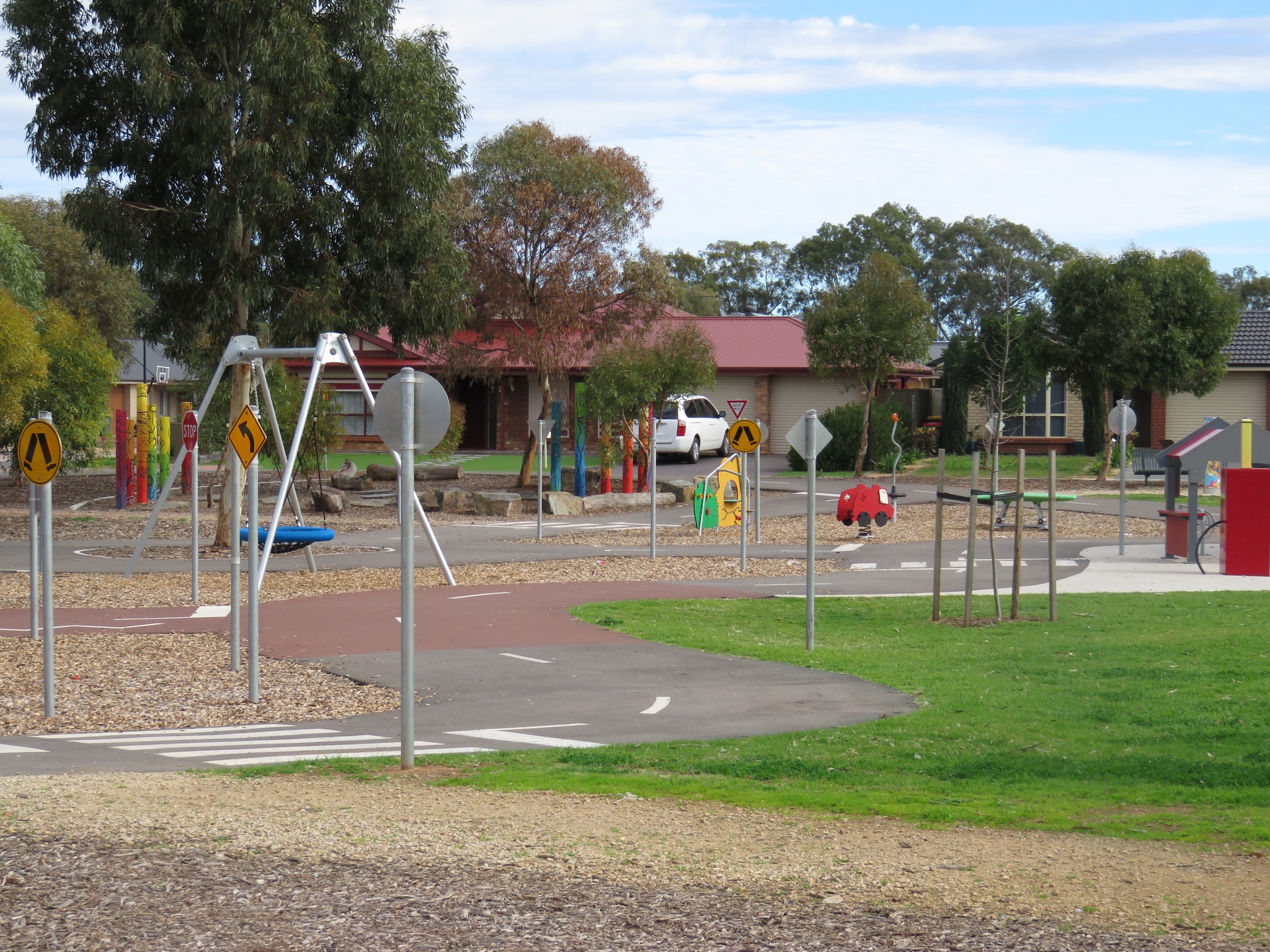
966, 490, 1077, 532
1131, 449, 1190, 486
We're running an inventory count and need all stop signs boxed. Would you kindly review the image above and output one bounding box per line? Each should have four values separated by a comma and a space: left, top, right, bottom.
181, 411, 199, 451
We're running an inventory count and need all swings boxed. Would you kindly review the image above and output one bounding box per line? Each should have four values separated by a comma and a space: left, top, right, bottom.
239, 357, 336, 554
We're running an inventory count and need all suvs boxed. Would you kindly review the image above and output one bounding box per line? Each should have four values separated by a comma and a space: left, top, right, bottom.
618, 392, 730, 464
